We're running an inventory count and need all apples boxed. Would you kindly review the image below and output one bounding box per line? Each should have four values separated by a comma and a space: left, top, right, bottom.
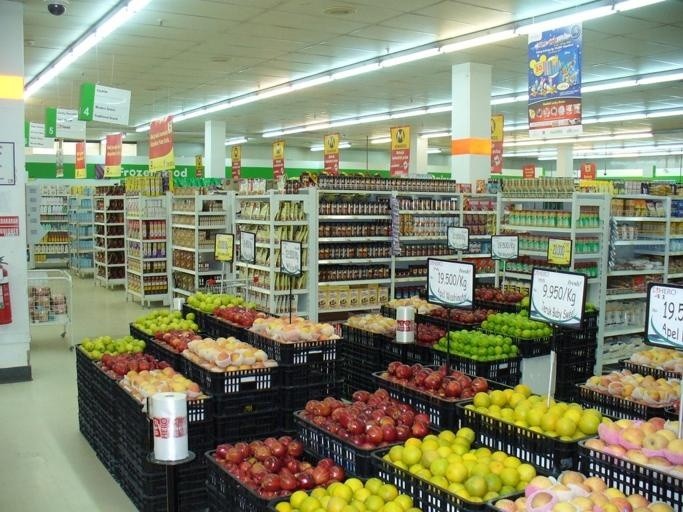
346, 286, 596, 359
205, 361, 683, 512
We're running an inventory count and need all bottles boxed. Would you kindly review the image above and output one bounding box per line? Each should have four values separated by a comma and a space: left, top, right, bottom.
501, 178, 575, 198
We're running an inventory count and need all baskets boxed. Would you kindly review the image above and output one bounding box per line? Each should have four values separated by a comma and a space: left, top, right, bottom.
204, 446, 341, 511
618, 357, 683, 381
293, 409, 441, 479
555, 308, 599, 406
371, 437, 487, 512
339, 320, 521, 410
573, 372, 682, 428
458, 395, 600, 480
577, 439, 683, 512
129, 303, 343, 440
75, 338, 213, 511
382, 288, 555, 358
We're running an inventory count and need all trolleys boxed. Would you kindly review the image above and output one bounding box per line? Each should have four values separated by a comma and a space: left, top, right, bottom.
27, 269, 74, 352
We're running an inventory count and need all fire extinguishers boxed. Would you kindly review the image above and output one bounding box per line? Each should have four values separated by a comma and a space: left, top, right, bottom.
0, 258, 11, 326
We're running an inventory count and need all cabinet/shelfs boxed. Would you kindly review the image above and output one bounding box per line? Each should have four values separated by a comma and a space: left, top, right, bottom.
316, 177, 683, 376
26, 178, 315, 326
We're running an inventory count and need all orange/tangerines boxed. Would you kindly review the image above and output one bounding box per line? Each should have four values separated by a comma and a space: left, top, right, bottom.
79, 292, 256, 359
